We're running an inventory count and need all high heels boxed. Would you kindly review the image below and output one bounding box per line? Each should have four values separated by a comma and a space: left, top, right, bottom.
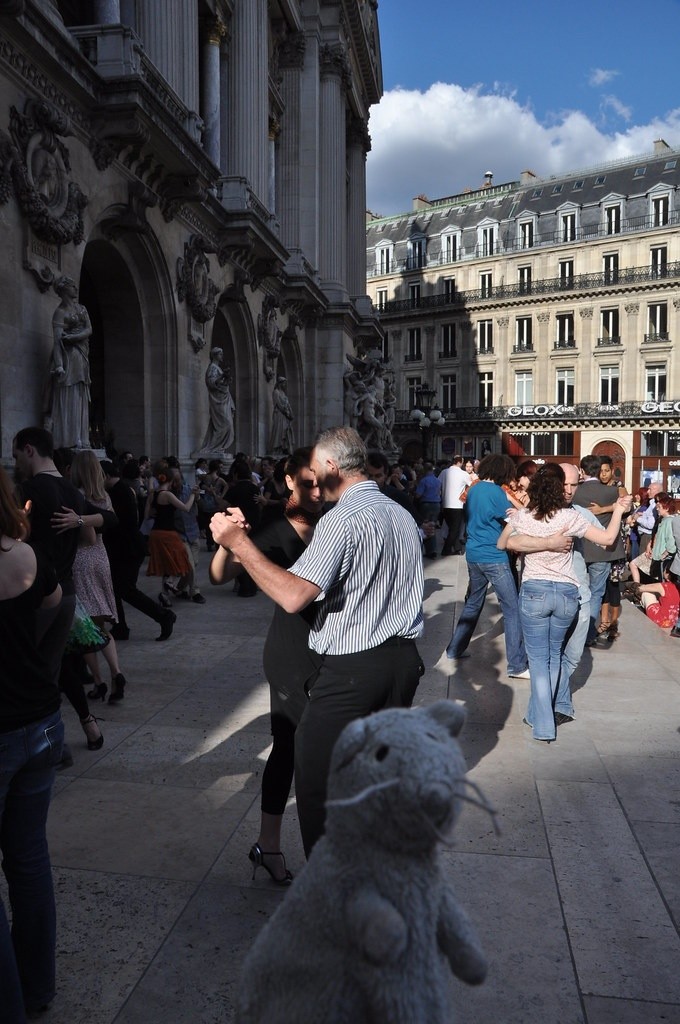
164, 581, 180, 593
87, 682, 107, 702
159, 593, 172, 608
248, 843, 293, 886
108, 673, 126, 701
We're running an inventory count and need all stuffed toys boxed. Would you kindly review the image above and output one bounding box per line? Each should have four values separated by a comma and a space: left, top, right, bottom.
239, 701, 489, 1024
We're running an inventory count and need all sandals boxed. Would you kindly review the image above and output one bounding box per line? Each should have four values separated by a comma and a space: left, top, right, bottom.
80, 714, 105, 751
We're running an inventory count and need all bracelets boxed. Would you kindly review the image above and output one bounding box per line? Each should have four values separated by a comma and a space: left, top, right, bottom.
78, 516, 83, 527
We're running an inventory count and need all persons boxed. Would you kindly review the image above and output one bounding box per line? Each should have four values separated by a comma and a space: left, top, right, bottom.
0, 423, 680, 1024
202, 347, 236, 452
46, 275, 92, 446
265, 377, 295, 454
346, 368, 397, 451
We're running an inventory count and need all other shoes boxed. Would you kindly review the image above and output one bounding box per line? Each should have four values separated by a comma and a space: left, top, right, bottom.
595, 622, 610, 637
456, 550, 463, 555
431, 552, 437, 560
459, 648, 471, 657
509, 668, 530, 679
553, 711, 574, 727
192, 593, 206, 603
607, 625, 618, 642
156, 609, 177, 641
110, 627, 130, 640
447, 551, 456, 555
670, 625, 680, 636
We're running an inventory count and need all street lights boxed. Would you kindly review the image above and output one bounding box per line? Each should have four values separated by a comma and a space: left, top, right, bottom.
409, 381, 446, 464
